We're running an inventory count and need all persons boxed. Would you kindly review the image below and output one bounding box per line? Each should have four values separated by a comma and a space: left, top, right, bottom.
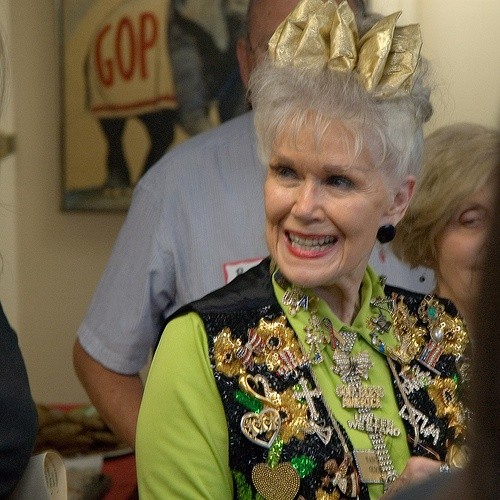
71, 0, 439, 451
79, 1, 270, 194
134, 0, 470, 500
391, 124, 500, 472
0, 304, 39, 500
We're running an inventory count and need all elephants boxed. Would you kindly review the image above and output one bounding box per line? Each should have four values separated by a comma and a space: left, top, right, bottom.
83, 0, 253, 196
